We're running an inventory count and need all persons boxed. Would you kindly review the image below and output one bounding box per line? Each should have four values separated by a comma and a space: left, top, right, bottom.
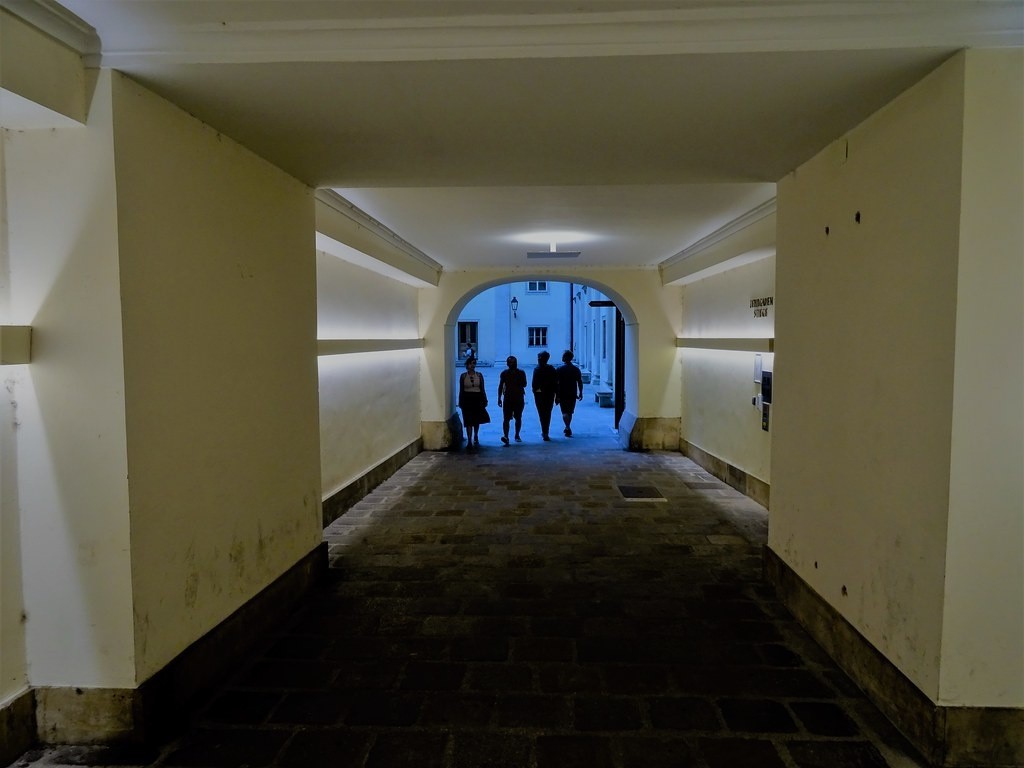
555, 350, 583, 436
463, 342, 474, 361
532, 351, 556, 441
498, 356, 527, 444
458, 357, 490, 448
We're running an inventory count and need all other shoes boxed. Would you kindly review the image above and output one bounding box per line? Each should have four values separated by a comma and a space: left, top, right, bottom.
541, 432, 550, 441
501, 436, 510, 444
564, 428, 572, 436
515, 436, 521, 442
466, 440, 480, 451
